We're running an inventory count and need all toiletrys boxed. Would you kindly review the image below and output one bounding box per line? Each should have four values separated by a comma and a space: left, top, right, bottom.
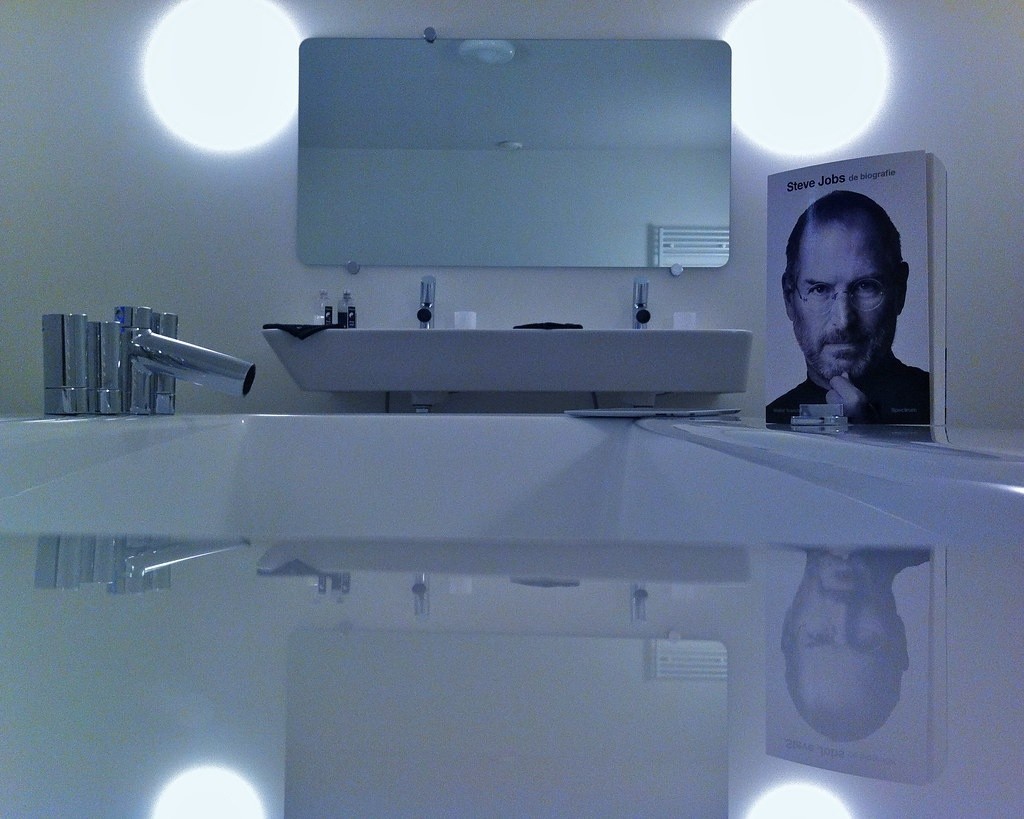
335, 288, 357, 328
313, 288, 335, 326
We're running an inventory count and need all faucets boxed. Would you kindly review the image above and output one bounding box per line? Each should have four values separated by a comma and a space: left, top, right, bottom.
632, 274, 654, 327
417, 274, 438, 327
411, 571, 436, 625
630, 583, 649, 623
145, 332, 257, 396
141, 539, 251, 573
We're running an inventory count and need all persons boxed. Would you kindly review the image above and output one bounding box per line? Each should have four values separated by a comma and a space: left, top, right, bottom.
778, 542, 935, 744
764, 190, 934, 426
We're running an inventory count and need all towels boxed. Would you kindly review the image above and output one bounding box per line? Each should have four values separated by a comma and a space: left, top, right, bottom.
509, 577, 581, 588
512, 322, 583, 329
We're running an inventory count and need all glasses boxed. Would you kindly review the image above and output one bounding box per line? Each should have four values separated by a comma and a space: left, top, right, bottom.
795, 265, 902, 315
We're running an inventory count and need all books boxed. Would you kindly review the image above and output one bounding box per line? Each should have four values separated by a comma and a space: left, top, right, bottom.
763, 540, 951, 787
763, 149, 949, 429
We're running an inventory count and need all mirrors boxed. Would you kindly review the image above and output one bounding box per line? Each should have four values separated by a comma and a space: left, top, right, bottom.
296, 36, 733, 266
280, 625, 734, 818
